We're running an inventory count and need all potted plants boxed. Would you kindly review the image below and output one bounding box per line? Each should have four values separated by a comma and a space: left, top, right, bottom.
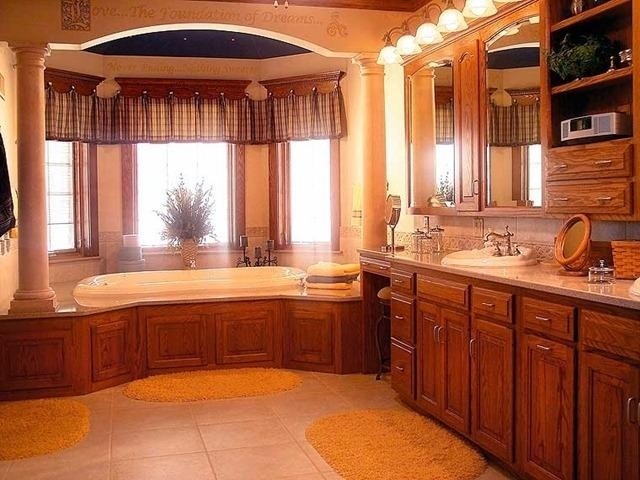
152, 173, 223, 269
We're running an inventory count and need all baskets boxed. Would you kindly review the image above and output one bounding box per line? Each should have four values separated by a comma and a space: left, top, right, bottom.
611, 239, 640, 281
568, 240, 612, 275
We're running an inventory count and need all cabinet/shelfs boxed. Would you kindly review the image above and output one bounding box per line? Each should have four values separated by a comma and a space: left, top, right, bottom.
390, 267, 640, 480
540, 0, 640, 220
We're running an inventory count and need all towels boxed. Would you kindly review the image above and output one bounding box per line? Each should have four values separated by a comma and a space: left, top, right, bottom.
304, 262, 361, 297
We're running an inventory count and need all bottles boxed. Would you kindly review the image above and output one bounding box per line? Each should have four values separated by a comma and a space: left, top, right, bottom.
589, 260, 616, 285
411, 225, 445, 255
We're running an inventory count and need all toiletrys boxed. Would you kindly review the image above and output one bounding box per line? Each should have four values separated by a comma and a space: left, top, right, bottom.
124, 235, 138, 247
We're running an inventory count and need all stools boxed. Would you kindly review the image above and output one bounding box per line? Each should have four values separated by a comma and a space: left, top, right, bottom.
375, 286, 391, 381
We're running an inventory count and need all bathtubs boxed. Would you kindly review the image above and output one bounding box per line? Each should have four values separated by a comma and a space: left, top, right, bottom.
72, 265, 307, 307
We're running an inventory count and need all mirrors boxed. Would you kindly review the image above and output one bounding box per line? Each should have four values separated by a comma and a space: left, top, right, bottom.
485, 13, 543, 207
408, 57, 456, 208
554, 214, 591, 276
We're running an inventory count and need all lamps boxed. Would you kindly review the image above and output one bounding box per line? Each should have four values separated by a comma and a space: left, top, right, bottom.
376, 0, 497, 67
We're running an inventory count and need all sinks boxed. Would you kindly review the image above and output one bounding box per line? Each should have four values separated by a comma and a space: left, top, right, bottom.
442, 246, 538, 266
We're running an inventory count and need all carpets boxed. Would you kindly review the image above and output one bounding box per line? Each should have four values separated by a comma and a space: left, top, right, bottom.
122, 367, 305, 403
305, 407, 488, 480
0, 398, 94, 461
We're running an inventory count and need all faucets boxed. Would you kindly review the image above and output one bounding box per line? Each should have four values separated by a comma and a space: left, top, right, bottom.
100, 282, 108, 288
482, 224, 514, 254
